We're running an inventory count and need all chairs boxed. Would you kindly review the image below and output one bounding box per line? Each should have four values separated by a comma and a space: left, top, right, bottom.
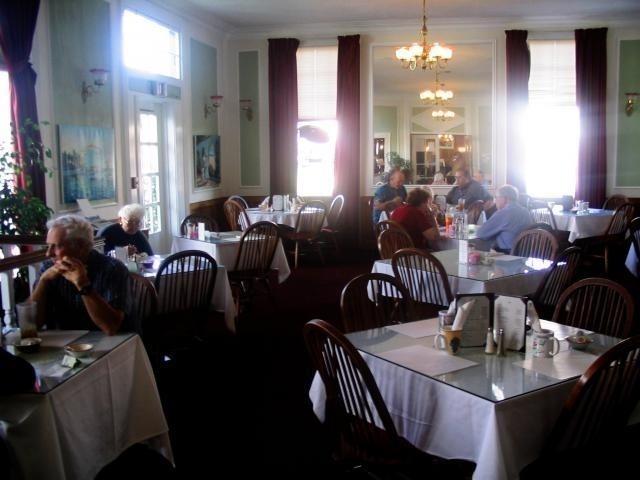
391, 248, 454, 317
223, 200, 251, 230
531, 221, 555, 233
511, 226, 559, 262
284, 200, 328, 268
179, 212, 219, 236
322, 193, 344, 249
529, 246, 594, 324
628, 217, 639, 260
154, 249, 219, 334
227, 220, 281, 322
338, 273, 415, 332
229, 195, 249, 208
124, 272, 161, 356
523, 337, 638, 479
551, 277, 637, 342
603, 194, 630, 211
584, 203, 633, 272
304, 317, 431, 479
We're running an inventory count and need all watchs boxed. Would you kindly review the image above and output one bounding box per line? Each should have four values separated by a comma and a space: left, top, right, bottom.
78, 283, 94, 296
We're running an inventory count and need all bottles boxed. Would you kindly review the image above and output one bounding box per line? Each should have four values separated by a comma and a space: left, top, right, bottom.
484, 327, 506, 358
444, 213, 464, 240
186, 222, 206, 241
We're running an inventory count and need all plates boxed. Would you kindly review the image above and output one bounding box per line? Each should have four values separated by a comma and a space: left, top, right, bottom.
488, 255, 526, 262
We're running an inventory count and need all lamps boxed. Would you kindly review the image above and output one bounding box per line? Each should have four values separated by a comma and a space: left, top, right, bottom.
420, 71, 455, 121
395, 0, 453, 71
205, 96, 224, 118
81, 68, 111, 104
624, 93, 639, 117
239, 99, 254, 122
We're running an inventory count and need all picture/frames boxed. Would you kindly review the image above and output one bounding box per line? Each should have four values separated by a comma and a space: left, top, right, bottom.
367, 39, 497, 191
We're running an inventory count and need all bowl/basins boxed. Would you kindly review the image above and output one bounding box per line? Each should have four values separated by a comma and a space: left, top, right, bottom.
64, 343, 93, 356
14, 337, 42, 354
566, 336, 595, 351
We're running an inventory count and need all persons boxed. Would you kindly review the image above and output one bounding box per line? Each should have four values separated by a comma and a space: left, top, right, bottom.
373, 168, 533, 255
15, 214, 137, 337
0, 348, 35, 480
95, 204, 154, 256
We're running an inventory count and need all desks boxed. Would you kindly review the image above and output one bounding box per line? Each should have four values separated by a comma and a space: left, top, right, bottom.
240, 204, 328, 247
113, 252, 239, 330
169, 229, 292, 281
317, 310, 627, 478
2, 324, 174, 477
536, 199, 613, 243
368, 247, 565, 309
435, 223, 485, 250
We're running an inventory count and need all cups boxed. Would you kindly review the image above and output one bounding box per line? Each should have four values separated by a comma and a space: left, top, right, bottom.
435, 327, 464, 355
458, 198, 465, 212
17, 301, 37, 341
530, 329, 560, 359
